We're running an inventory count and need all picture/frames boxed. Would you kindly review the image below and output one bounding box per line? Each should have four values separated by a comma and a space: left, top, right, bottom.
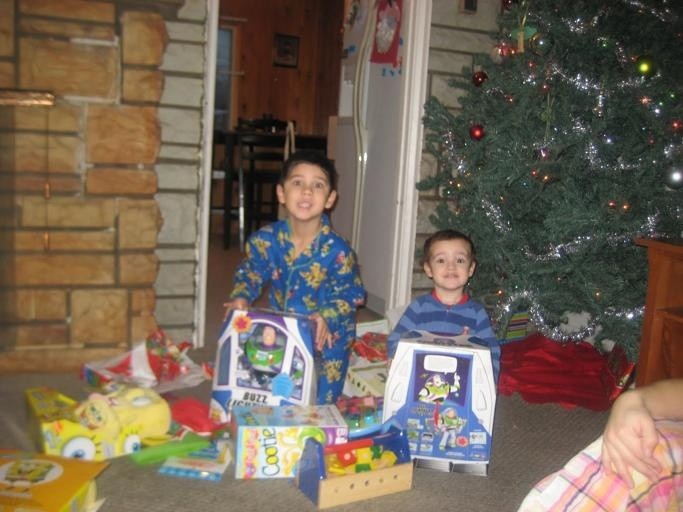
268, 30, 300, 70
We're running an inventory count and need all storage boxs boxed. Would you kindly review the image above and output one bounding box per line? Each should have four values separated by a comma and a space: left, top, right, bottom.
223, 402, 347, 482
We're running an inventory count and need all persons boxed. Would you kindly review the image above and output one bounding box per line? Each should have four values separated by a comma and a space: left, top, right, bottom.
601, 377, 683, 490
223, 147, 366, 405
386, 229, 501, 395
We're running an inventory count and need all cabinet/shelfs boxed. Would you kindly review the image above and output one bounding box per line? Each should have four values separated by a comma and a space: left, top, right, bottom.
629, 237, 682, 390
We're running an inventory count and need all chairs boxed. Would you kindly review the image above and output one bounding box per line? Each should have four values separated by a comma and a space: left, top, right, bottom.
207, 114, 329, 252
